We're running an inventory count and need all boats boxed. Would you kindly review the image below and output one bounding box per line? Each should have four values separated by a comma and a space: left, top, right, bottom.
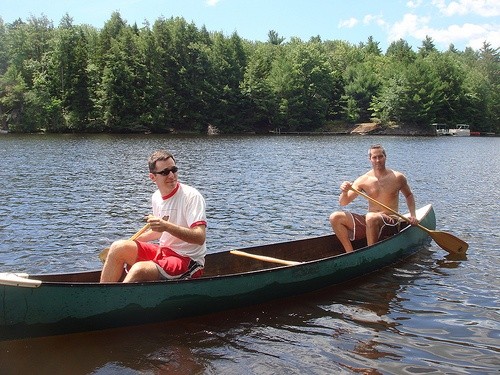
430, 123, 472, 137
0, 204, 438, 341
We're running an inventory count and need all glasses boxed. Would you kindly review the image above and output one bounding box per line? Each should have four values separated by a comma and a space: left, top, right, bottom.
153, 166, 179, 176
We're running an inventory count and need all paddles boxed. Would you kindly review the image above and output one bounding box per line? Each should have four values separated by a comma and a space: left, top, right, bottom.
98, 214, 156, 265
350, 186, 470, 257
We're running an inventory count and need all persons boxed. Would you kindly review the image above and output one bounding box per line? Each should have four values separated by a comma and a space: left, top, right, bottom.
100, 151, 208, 283
329, 145, 419, 253
